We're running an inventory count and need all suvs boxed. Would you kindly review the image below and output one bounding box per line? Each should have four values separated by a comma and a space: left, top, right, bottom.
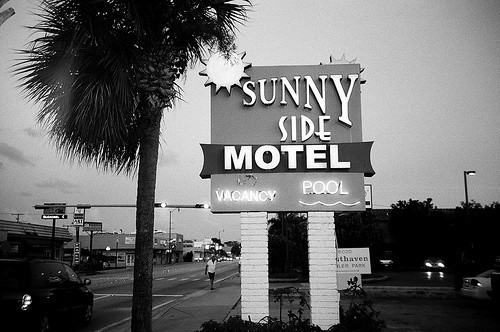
0, 257, 95, 332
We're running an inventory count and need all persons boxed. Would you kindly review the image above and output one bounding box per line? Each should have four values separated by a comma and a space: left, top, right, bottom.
205, 256, 217, 290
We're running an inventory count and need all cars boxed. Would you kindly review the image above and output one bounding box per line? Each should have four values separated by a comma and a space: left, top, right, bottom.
375, 251, 396, 271
462, 268, 500, 302
216, 254, 241, 262
423, 255, 448, 272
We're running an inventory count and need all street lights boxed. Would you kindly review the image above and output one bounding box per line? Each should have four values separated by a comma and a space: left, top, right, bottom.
219, 229, 224, 254
464, 170, 476, 242
168, 207, 180, 264
115, 238, 119, 269
